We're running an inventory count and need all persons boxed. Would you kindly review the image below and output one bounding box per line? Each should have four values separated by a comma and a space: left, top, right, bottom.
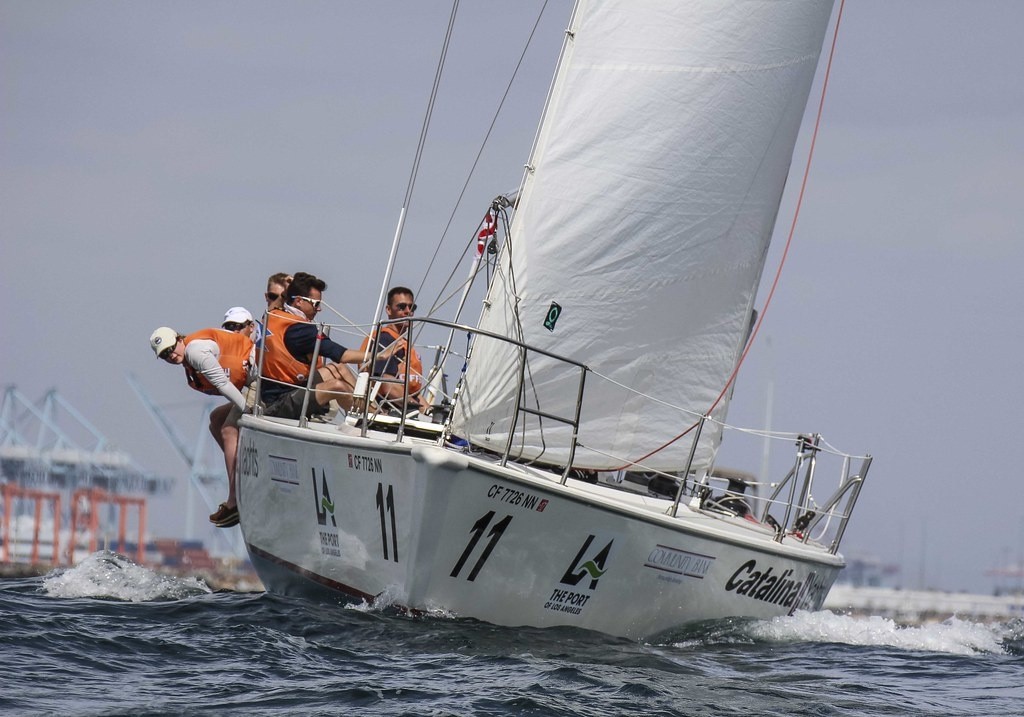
357, 286, 435, 421
149, 327, 259, 528
209, 271, 407, 452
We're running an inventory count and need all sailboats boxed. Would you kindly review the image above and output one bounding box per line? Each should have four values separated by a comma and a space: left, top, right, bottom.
232, 1, 872, 644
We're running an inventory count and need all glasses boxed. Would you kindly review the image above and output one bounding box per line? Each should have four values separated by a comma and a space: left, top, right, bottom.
267, 292, 284, 300
292, 296, 321, 308
160, 341, 177, 359
391, 303, 417, 311
225, 322, 249, 331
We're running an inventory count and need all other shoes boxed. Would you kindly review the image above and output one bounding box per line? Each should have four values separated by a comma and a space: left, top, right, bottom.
209, 503, 239, 523
217, 516, 239, 527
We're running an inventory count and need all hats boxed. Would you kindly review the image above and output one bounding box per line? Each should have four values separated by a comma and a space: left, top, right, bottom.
222, 307, 253, 328
150, 327, 180, 359
